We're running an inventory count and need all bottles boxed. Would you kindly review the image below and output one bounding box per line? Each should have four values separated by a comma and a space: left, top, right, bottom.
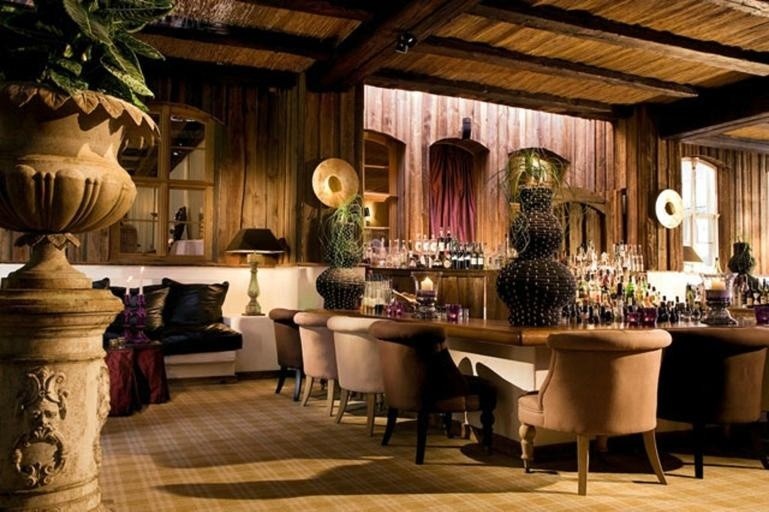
752, 279, 760, 306
714, 258, 722, 274
421, 233, 429, 269
554, 240, 624, 268
436, 228, 445, 267
483, 239, 510, 269
740, 275, 748, 307
463, 240, 471, 272
415, 233, 422, 263
407, 238, 413, 267
399, 239, 407, 266
691, 291, 703, 321
669, 295, 681, 325
732, 277, 740, 309
385, 239, 396, 269
564, 265, 670, 328
444, 230, 452, 268
451, 244, 458, 271
744, 281, 754, 309
625, 244, 644, 272
685, 284, 695, 311
759, 278, 768, 304
429, 234, 437, 268
457, 241, 464, 271
469, 241, 477, 270
477, 241, 483, 270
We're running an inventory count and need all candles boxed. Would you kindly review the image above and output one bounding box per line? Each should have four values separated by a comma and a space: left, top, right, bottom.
137, 277, 151, 302
122, 274, 134, 298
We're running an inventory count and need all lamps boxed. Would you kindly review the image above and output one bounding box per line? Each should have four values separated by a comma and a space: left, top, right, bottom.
224, 227, 287, 315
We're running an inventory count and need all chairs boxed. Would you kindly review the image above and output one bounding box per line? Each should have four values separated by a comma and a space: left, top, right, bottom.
265, 305, 308, 404
661, 325, 769, 482
326, 314, 403, 435
290, 310, 355, 417
372, 318, 500, 467
514, 326, 675, 496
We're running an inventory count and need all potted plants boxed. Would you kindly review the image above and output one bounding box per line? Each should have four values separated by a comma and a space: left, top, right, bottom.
484, 144, 579, 326
0, 0, 182, 512
317, 193, 374, 313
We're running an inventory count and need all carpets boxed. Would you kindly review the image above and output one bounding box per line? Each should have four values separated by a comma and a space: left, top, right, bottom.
0, 371, 769, 510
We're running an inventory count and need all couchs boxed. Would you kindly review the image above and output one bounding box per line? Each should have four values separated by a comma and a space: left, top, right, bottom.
89, 281, 244, 384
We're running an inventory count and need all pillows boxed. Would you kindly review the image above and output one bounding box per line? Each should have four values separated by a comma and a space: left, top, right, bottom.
162, 277, 227, 325
107, 285, 172, 337
92, 278, 109, 291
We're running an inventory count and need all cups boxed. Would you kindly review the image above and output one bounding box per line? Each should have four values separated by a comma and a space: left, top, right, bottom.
444, 303, 460, 323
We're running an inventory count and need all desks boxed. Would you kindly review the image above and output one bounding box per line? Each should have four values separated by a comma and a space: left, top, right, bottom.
222, 314, 281, 380
